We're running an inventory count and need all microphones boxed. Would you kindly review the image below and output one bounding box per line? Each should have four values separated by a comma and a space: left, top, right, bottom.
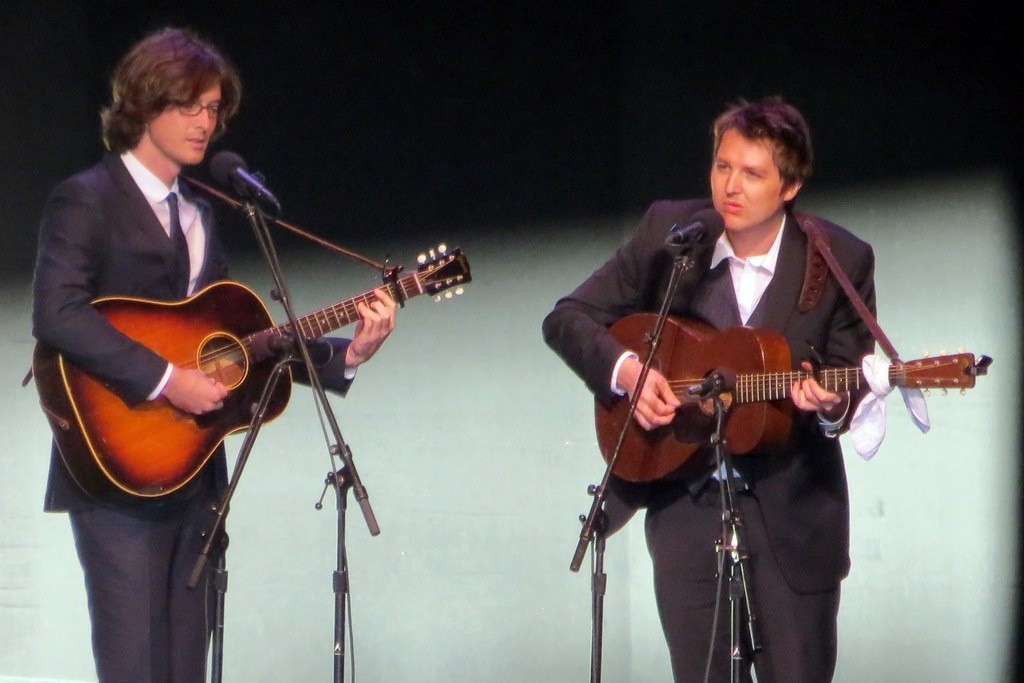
208, 151, 279, 214
267, 333, 332, 369
665, 208, 726, 246
685, 366, 735, 400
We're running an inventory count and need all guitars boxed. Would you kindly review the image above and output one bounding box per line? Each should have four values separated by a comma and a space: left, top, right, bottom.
593, 313, 995, 488
31, 240, 473, 511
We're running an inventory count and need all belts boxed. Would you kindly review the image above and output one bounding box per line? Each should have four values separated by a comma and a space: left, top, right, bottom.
705, 477, 749, 492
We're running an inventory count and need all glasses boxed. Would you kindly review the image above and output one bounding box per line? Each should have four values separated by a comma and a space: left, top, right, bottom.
173, 98, 234, 121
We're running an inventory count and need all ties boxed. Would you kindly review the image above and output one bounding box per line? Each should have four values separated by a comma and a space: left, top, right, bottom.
166, 192, 191, 299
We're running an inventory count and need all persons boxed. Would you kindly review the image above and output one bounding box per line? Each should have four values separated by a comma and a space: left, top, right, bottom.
542, 98, 878, 682
31, 28, 396, 683
